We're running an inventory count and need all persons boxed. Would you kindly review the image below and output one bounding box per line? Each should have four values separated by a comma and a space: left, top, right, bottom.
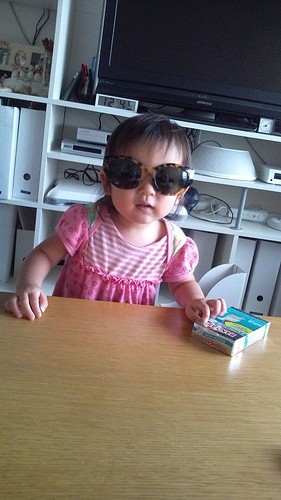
4, 113, 228, 326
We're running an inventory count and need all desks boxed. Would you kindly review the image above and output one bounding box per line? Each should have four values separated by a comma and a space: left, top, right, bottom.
0, 291, 281, 500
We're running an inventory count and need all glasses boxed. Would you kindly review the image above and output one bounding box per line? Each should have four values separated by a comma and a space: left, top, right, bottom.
102, 155, 195, 196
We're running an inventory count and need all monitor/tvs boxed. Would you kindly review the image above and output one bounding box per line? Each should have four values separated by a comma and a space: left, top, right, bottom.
93, 0, 281, 120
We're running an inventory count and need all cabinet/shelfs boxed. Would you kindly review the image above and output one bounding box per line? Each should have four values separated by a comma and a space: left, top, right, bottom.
0, 1, 281, 316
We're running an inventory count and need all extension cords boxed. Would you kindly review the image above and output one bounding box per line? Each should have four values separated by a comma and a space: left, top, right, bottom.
216, 204, 269, 223
